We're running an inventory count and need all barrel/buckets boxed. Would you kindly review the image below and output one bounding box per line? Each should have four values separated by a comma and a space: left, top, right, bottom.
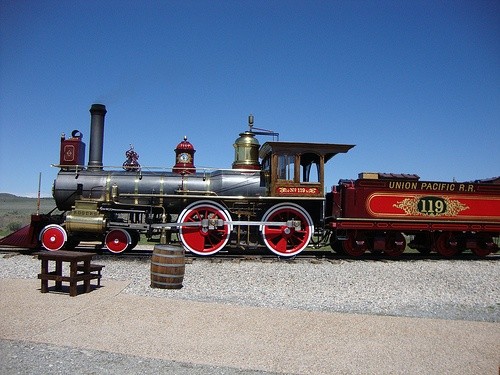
149, 244, 186, 290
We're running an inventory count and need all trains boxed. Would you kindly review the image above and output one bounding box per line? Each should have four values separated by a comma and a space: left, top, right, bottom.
1, 102, 499, 257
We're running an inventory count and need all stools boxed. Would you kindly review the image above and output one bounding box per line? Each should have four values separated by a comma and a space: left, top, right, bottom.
37, 250, 96, 297
69, 264, 105, 289
